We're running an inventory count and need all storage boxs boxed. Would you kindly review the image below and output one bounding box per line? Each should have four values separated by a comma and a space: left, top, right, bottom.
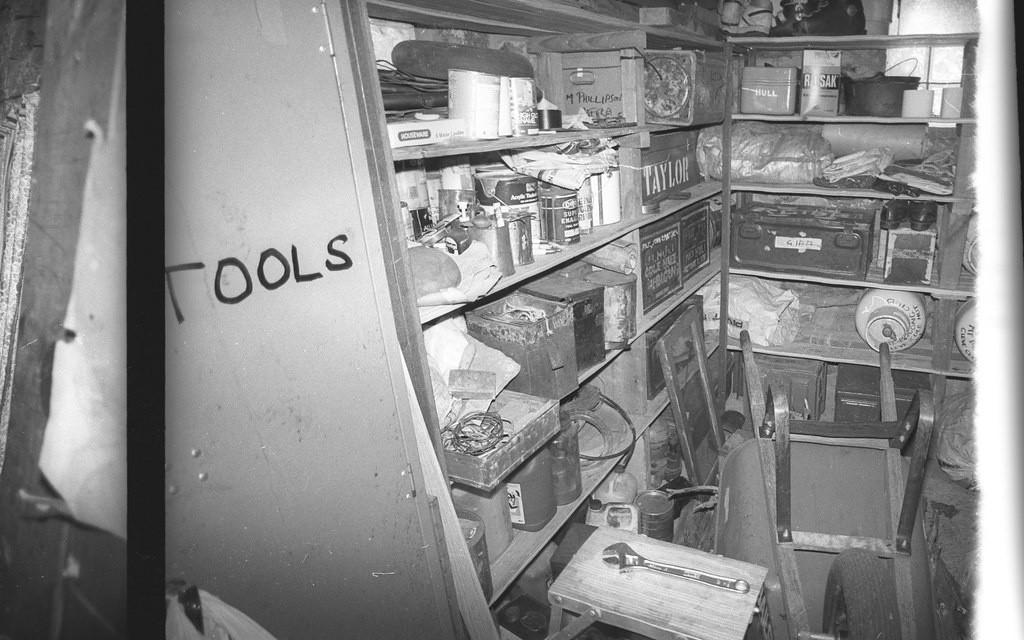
640, 128, 705, 208
738, 65, 799, 116
531, 44, 727, 127
881, 223, 939, 287
465, 289, 582, 402
523, 276, 610, 374
636, 200, 719, 317
729, 202, 878, 280
798, 47, 842, 119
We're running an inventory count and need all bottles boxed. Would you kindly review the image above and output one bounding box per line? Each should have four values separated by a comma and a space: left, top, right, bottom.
451, 446, 558, 564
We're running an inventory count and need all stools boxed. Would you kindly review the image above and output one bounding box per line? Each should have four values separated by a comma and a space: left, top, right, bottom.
546, 522, 771, 640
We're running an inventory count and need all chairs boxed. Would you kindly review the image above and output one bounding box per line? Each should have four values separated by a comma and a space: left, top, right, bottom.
736, 324, 934, 640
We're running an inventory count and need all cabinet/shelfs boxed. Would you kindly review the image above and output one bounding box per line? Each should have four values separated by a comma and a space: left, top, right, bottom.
339, 0, 731, 640
717, 30, 985, 442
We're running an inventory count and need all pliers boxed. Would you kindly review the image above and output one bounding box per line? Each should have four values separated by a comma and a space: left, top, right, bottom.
664, 191, 692, 201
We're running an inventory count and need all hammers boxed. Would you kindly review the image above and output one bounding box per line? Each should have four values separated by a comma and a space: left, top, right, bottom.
641, 191, 668, 214
